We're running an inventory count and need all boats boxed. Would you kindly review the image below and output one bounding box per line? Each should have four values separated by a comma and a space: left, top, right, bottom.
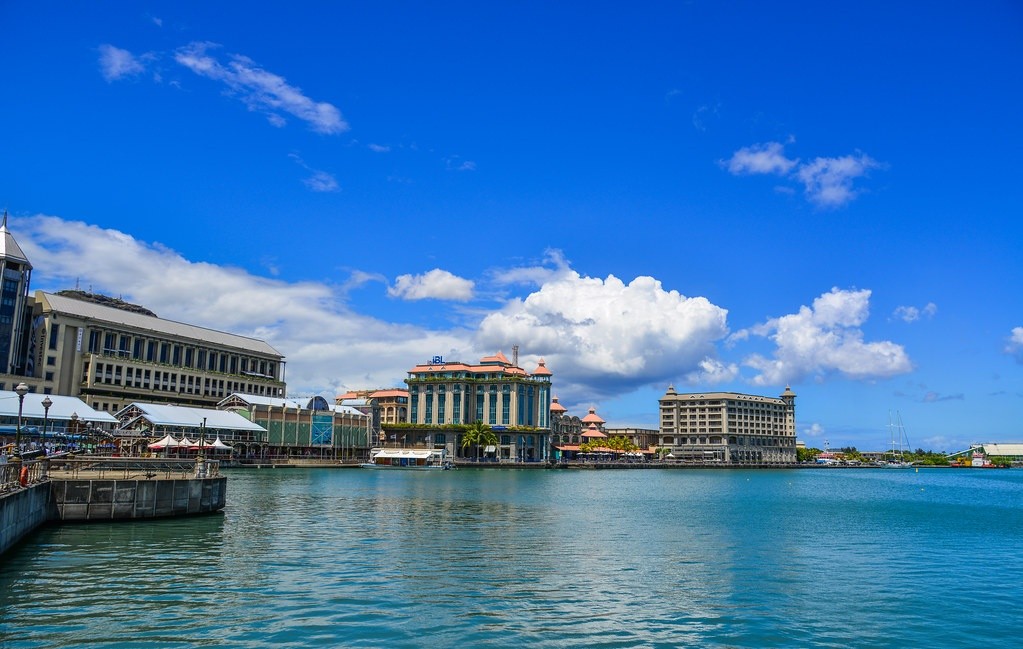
362, 449, 446, 470
948, 449, 1004, 468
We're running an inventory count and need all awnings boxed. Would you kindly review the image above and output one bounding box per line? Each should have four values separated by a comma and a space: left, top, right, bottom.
485, 446, 496, 452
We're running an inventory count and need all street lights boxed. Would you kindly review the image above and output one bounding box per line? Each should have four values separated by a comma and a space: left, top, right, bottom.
96, 426, 114, 453
86, 421, 92, 451
71, 411, 78, 453
41, 396, 54, 456
79, 417, 86, 450
13, 383, 29, 457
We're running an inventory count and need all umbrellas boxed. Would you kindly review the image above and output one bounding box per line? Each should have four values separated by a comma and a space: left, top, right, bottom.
577, 452, 612, 456
620, 451, 645, 459
666, 453, 674, 460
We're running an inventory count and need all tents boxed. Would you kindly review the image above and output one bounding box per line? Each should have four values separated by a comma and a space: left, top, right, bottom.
148, 434, 234, 450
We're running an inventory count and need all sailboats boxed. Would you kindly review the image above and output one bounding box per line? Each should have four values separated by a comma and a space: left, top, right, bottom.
878, 408, 915, 469
